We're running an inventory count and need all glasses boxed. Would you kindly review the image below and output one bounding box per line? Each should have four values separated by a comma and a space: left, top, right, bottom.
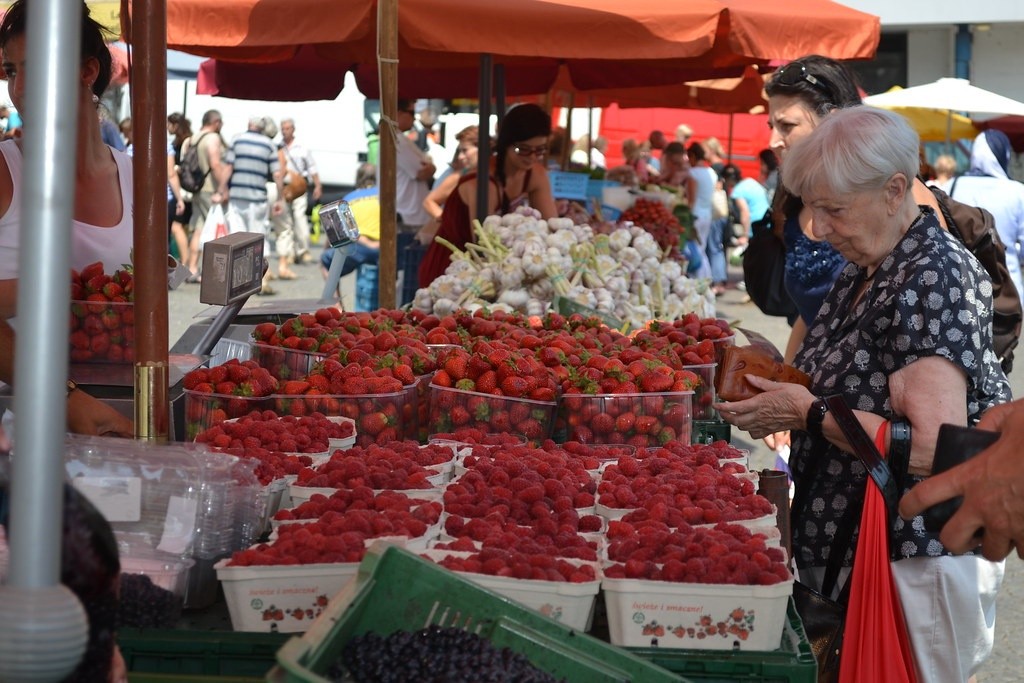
400, 109, 415, 117
771, 63, 826, 90
511, 144, 548, 157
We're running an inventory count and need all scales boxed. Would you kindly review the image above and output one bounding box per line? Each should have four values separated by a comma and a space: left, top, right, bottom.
70, 231, 269, 443
168, 199, 360, 353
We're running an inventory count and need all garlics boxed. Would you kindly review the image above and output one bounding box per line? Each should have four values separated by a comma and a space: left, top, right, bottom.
411, 206, 719, 328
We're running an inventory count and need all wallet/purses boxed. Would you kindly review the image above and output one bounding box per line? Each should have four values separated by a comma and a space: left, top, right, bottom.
923, 423, 1000, 536
719, 346, 808, 401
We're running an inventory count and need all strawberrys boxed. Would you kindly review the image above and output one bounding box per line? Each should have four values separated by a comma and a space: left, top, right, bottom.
184, 308, 734, 450
613, 197, 685, 257
69, 261, 134, 364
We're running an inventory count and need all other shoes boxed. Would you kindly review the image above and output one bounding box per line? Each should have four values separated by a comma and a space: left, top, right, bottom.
258, 283, 276, 296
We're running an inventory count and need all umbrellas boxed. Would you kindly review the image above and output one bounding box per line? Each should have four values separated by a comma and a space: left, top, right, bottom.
0, 0, 1024, 149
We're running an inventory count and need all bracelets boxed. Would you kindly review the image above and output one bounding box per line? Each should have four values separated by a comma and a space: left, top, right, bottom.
66, 379, 76, 399
804, 398, 829, 447
217, 191, 223, 196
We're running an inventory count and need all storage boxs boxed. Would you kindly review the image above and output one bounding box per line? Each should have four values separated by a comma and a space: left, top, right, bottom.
355, 233, 429, 312
190, 416, 819, 683
548, 170, 623, 222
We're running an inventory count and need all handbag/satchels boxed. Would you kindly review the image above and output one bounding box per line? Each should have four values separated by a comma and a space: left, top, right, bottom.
283, 170, 307, 202
790, 580, 847, 683
200, 203, 228, 242
838, 421, 917, 683
740, 216, 797, 318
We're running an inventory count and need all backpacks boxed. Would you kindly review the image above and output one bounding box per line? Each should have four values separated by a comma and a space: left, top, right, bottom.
176, 132, 212, 193
928, 184, 1023, 375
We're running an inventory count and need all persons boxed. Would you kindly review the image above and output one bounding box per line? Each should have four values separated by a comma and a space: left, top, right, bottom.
0, 55, 1024, 683
0, 0, 170, 331
